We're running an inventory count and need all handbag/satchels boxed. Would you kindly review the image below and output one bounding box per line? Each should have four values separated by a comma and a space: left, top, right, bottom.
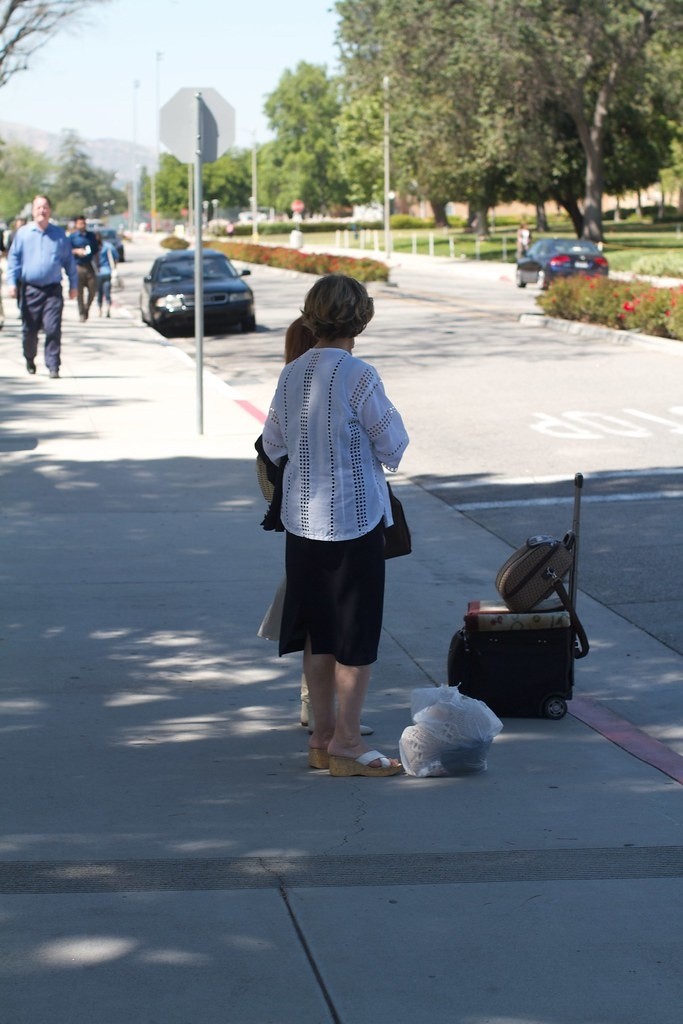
399, 686, 503, 779
111, 270, 120, 287
494, 530, 589, 659
383, 481, 411, 560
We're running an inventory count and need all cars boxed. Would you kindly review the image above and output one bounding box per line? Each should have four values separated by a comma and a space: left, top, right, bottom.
86, 218, 125, 262
514, 236, 611, 292
138, 248, 257, 337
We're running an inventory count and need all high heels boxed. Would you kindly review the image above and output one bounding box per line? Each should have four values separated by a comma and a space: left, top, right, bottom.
329, 749, 403, 778
309, 748, 330, 768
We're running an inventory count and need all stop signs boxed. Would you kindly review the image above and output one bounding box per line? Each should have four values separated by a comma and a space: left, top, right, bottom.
291, 200, 304, 212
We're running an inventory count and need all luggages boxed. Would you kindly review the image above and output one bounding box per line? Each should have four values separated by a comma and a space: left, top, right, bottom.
446, 472, 584, 720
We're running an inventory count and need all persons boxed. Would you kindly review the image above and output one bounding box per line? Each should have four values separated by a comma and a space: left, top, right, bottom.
8, 194, 79, 379
261, 274, 404, 779
63, 215, 121, 322
0, 215, 33, 330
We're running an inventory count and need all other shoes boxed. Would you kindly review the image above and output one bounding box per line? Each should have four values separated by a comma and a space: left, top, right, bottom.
80, 312, 87, 321
49, 369, 58, 378
309, 725, 373, 737
26, 357, 35, 374
